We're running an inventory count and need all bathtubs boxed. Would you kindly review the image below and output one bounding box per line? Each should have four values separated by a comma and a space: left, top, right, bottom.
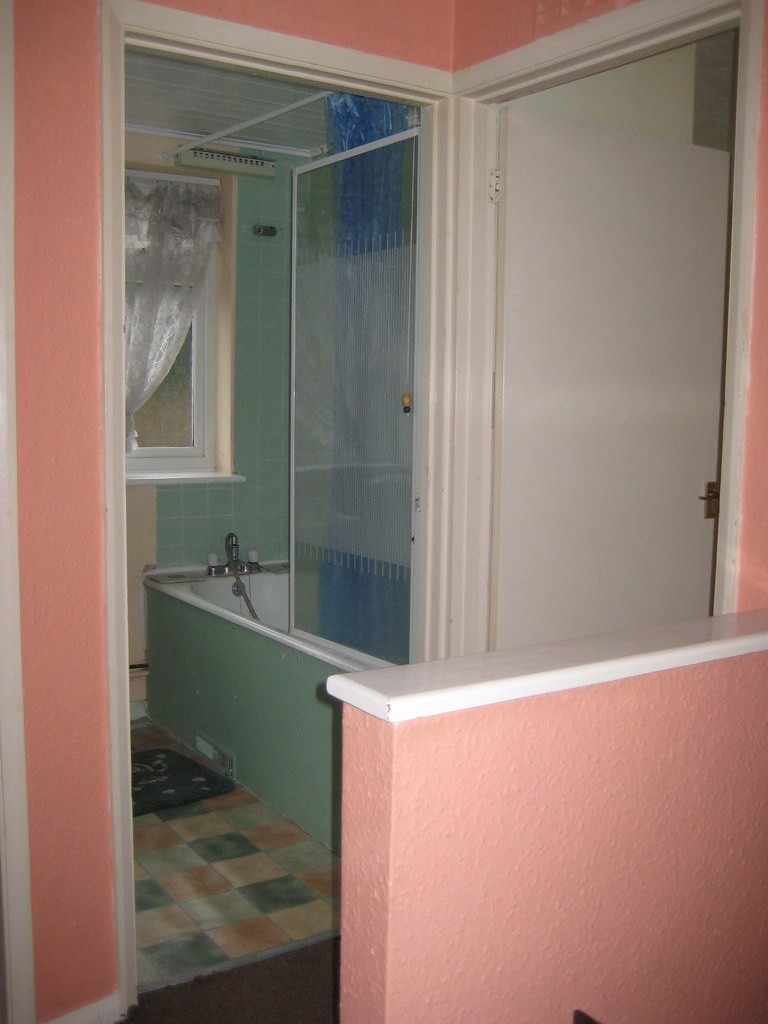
143, 553, 400, 855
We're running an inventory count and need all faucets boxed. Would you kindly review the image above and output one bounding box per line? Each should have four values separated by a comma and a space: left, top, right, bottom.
226, 531, 242, 567
